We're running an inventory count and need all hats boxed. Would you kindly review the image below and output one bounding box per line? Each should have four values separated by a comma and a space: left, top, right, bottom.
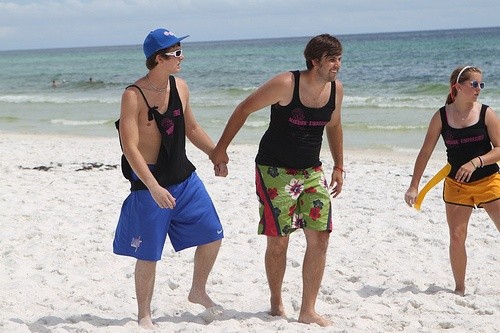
142, 29, 190, 58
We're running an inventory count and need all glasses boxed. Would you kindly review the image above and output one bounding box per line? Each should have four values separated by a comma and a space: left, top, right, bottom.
165, 49, 182, 57
460, 80, 485, 91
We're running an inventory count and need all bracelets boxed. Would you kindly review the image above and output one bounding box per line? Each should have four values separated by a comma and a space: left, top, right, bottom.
478, 156, 484, 168
471, 160, 477, 168
333, 166, 346, 178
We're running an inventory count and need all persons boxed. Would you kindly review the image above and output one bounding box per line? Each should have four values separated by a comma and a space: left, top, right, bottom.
211, 33, 343, 327
113, 29, 228, 328
405, 65, 500, 297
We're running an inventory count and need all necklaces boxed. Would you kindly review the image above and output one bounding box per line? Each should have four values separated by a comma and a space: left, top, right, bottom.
145, 76, 168, 92
453, 103, 469, 121
